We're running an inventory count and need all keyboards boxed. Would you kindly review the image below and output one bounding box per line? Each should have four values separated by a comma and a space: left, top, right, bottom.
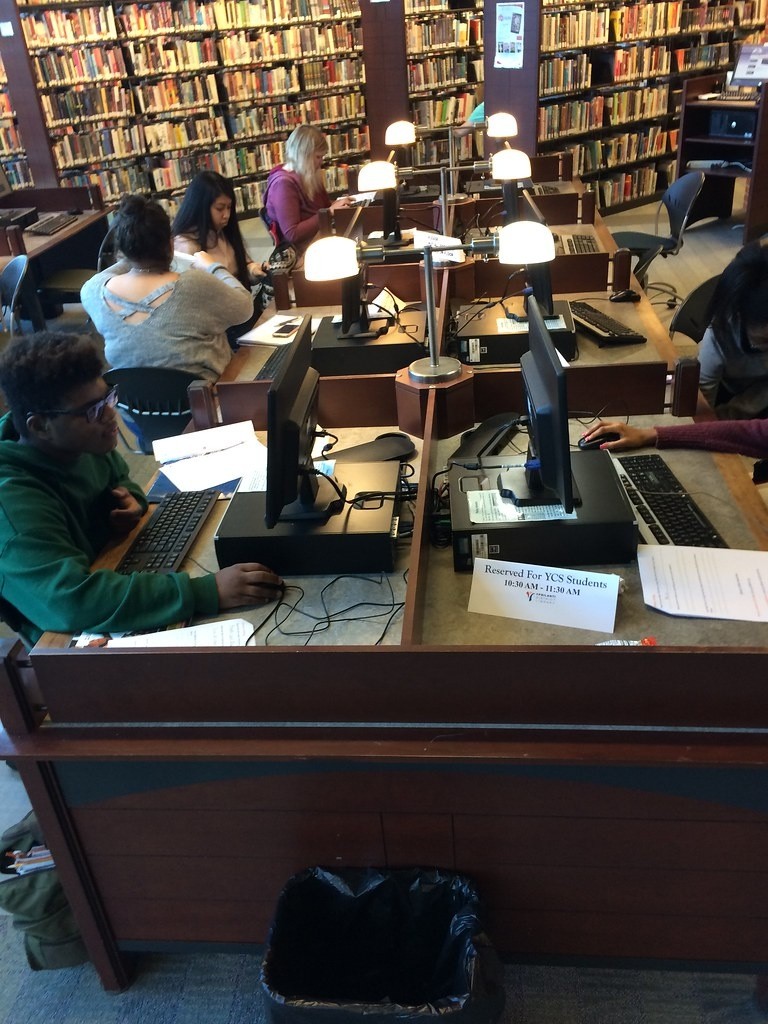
253, 342, 293, 381
24, 213, 77, 235
535, 185, 559, 196
560, 235, 600, 255
611, 453, 730, 551
716, 91, 761, 101
114, 490, 221, 575
568, 302, 647, 348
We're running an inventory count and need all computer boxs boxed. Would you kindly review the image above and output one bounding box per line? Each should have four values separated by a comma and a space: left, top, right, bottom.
214, 459, 404, 576
465, 176, 536, 199
459, 300, 576, 365
310, 310, 427, 377
446, 448, 638, 573
0, 207, 39, 230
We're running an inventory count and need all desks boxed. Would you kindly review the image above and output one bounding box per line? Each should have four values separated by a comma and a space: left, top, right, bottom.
0, 152, 768, 996
674, 74, 768, 246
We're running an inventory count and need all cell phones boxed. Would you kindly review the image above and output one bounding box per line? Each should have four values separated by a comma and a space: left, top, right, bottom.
273, 323, 301, 338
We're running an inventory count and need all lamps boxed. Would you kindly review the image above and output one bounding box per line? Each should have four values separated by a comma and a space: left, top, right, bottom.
385, 112, 518, 204
305, 221, 555, 383
358, 148, 531, 266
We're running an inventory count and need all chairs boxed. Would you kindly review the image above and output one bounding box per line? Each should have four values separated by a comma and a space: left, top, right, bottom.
102, 366, 206, 455
632, 244, 663, 284
0, 255, 30, 337
611, 170, 705, 307
98, 226, 116, 272
669, 274, 722, 344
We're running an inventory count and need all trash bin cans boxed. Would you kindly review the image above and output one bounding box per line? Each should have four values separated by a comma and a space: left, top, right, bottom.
261, 859, 485, 1024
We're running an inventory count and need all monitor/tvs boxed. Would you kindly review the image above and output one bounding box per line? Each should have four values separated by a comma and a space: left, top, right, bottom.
0, 164, 14, 198
506, 189, 560, 321
496, 295, 582, 515
379, 147, 420, 247
729, 45, 768, 86
335, 207, 389, 340
502, 178, 518, 225
263, 313, 346, 529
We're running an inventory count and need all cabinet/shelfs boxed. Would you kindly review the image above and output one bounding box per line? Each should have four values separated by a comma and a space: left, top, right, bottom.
0, 0, 767, 245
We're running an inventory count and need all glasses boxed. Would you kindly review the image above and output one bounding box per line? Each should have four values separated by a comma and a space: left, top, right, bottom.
25, 383, 121, 424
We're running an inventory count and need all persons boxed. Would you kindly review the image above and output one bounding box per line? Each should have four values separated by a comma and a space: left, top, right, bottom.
169, 171, 269, 350
0, 330, 287, 646
262, 124, 356, 252
454, 83, 485, 138
698, 238, 768, 416
582, 418, 768, 460
81, 195, 255, 384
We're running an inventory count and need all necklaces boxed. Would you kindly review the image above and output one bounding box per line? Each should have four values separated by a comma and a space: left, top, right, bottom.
130, 267, 151, 273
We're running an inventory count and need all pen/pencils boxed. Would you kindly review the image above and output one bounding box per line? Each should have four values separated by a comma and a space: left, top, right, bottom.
273, 318, 298, 327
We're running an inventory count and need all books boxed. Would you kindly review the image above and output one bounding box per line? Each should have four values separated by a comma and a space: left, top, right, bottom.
0, 0, 371, 226
403, 0, 768, 210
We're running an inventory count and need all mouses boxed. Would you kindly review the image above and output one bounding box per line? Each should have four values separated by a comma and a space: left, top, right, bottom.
609, 289, 641, 302
255, 580, 284, 593
552, 232, 559, 242
67, 208, 83, 215
